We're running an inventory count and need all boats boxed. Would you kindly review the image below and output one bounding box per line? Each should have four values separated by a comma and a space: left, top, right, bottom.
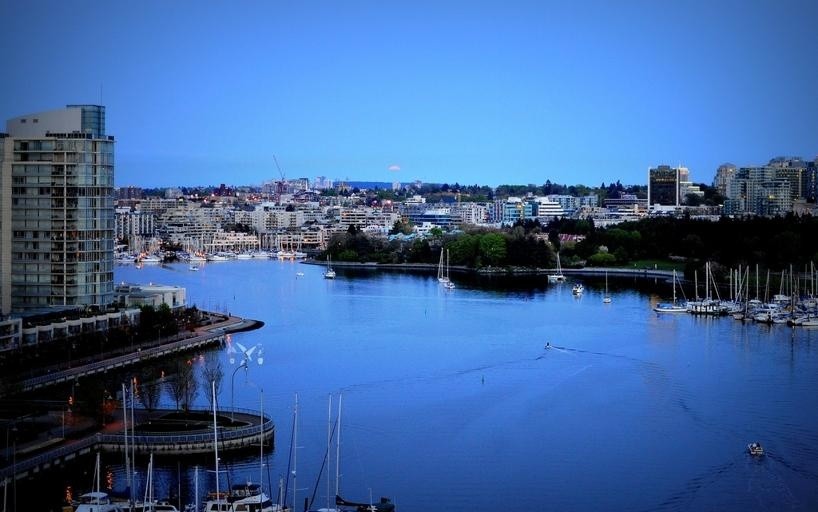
187, 266, 200, 273
296, 271, 303, 277
545, 339, 551, 351
568, 281, 586, 298
746, 441, 764, 458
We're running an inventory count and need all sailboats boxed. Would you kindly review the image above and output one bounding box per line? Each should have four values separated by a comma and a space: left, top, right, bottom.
82, 371, 395, 511
603, 268, 612, 303
115, 232, 309, 264
437, 247, 456, 290
323, 252, 335, 279
653, 259, 817, 328
549, 251, 566, 281
